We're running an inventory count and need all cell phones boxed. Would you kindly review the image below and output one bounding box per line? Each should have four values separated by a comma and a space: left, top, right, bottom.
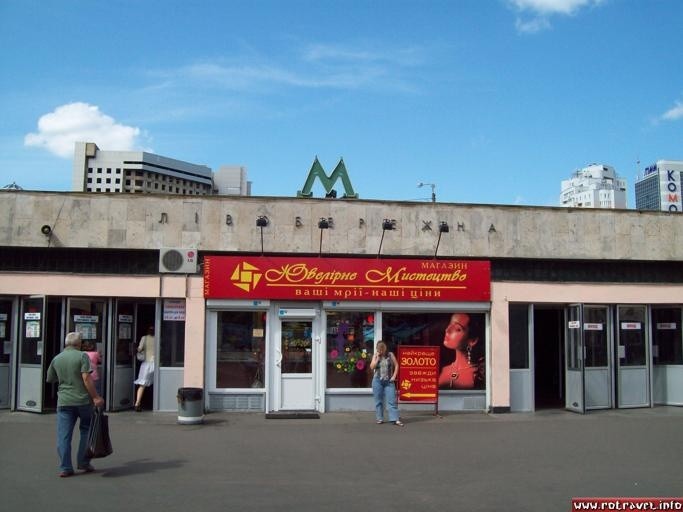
374, 350, 384, 360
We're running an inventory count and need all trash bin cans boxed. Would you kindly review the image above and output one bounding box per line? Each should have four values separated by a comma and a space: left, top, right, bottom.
176, 387, 205, 425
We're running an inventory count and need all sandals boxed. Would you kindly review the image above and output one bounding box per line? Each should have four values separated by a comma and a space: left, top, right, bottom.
134, 401, 142, 412
394, 420, 404, 427
376, 419, 384, 424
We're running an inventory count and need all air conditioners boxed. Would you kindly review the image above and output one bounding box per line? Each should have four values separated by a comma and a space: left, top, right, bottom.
157, 246, 198, 277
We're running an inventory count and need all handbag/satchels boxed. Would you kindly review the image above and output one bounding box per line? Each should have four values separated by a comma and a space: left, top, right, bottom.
83, 411, 114, 460
136, 350, 146, 362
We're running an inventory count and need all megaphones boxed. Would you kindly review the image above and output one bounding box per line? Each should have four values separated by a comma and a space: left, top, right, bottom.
41, 225, 52, 236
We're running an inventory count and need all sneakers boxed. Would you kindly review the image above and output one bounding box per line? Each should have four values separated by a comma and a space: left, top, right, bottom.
77, 463, 96, 472
59, 470, 76, 477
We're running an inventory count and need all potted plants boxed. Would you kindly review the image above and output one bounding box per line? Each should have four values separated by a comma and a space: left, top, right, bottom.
281, 336, 311, 363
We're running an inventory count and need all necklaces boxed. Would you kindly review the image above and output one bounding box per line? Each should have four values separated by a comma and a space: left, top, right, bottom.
448, 361, 478, 389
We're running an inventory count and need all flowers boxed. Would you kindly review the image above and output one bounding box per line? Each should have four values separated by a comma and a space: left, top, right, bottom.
329, 346, 370, 374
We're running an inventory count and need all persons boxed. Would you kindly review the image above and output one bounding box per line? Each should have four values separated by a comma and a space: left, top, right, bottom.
46, 331, 105, 477
81, 340, 102, 395
370, 341, 404, 428
133, 326, 156, 411
436, 312, 486, 389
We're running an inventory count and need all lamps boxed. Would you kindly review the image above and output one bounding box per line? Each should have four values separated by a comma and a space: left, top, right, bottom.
433, 220, 450, 259
255, 214, 268, 253
316, 217, 330, 255
377, 218, 394, 258
